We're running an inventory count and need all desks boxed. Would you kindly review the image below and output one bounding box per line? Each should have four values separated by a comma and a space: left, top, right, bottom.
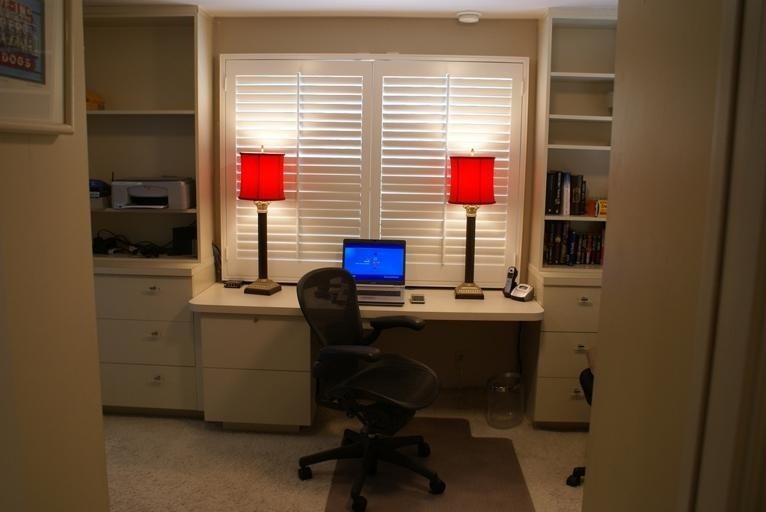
184, 278, 545, 435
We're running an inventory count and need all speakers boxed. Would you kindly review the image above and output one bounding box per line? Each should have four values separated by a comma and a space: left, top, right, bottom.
173, 227, 193, 255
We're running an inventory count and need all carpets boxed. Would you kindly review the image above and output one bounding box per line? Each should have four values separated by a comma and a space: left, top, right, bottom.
319, 416, 534, 512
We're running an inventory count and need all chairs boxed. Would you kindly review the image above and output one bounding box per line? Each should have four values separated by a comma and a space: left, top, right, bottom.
294, 265, 446, 511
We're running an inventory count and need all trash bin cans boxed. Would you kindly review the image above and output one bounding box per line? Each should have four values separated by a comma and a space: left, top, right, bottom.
486, 371, 524, 430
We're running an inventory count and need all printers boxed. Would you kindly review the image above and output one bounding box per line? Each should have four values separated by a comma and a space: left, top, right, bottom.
111, 177, 197, 210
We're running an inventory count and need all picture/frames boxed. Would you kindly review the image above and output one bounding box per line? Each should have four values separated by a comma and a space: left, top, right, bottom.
0, 2, 76, 137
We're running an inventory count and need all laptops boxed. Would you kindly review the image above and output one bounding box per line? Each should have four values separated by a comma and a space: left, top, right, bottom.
342, 238, 405, 305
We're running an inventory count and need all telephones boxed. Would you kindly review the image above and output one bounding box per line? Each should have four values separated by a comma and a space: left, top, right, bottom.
504, 266, 534, 301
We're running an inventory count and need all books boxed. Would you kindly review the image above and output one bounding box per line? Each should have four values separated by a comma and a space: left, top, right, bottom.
545, 170, 607, 269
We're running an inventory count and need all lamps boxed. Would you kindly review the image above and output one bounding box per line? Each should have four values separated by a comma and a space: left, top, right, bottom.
442, 146, 497, 301
235, 144, 290, 295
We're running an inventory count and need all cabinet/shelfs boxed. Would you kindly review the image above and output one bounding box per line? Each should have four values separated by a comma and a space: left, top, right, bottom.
512, 12, 621, 430
84, 3, 216, 414
